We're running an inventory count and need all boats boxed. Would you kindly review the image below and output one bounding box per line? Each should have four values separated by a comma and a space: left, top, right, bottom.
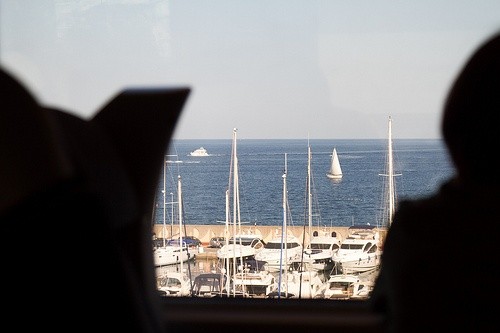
187, 145, 210, 157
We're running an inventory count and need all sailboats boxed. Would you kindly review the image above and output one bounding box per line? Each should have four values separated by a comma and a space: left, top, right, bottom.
158, 113, 407, 301
